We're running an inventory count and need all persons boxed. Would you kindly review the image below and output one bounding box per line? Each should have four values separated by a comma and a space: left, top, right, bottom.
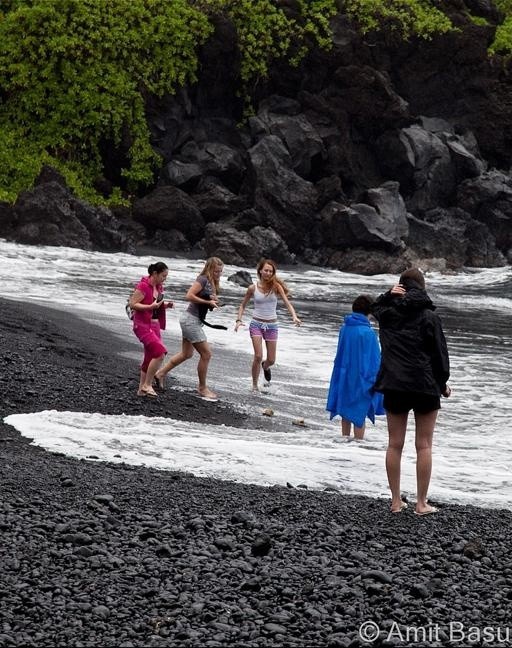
327, 291, 385, 439
234, 258, 303, 392
129, 262, 175, 397
370, 266, 449, 516
154, 253, 223, 402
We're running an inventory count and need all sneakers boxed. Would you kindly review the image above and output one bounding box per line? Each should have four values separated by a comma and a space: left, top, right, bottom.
261, 362, 271, 381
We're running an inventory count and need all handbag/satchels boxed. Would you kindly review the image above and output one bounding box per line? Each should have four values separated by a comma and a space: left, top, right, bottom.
126, 299, 134, 320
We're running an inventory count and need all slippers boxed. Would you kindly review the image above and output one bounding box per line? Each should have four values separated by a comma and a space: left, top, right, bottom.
393, 502, 408, 513
414, 506, 439, 515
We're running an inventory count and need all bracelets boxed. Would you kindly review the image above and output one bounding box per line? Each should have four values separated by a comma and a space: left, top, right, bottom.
236, 319, 242, 323
